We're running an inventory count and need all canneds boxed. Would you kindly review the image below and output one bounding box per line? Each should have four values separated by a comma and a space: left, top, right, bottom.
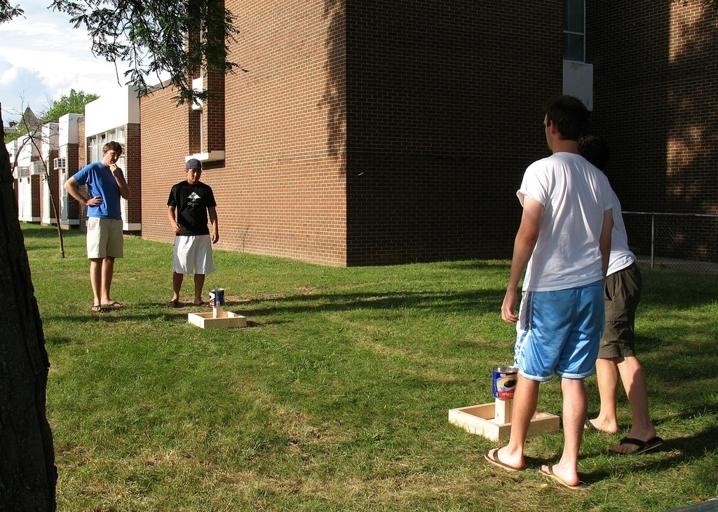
209, 287, 225, 308
492, 365, 519, 400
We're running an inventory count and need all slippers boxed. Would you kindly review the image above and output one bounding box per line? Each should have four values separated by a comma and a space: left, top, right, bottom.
484, 445, 526, 472
539, 464, 583, 490
194, 300, 205, 305
91, 302, 124, 312
582, 419, 616, 433
609, 435, 665, 457
169, 299, 179, 307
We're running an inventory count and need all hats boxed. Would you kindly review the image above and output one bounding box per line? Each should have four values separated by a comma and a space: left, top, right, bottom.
185, 159, 202, 170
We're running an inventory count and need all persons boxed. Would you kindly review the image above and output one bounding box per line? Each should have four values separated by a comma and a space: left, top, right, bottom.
481, 95, 615, 493
62, 141, 131, 313
166, 157, 220, 306
575, 133, 665, 459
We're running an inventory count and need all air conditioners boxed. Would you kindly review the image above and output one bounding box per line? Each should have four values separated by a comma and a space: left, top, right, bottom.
54, 158, 65, 169
30, 161, 46, 176
13, 167, 29, 179
